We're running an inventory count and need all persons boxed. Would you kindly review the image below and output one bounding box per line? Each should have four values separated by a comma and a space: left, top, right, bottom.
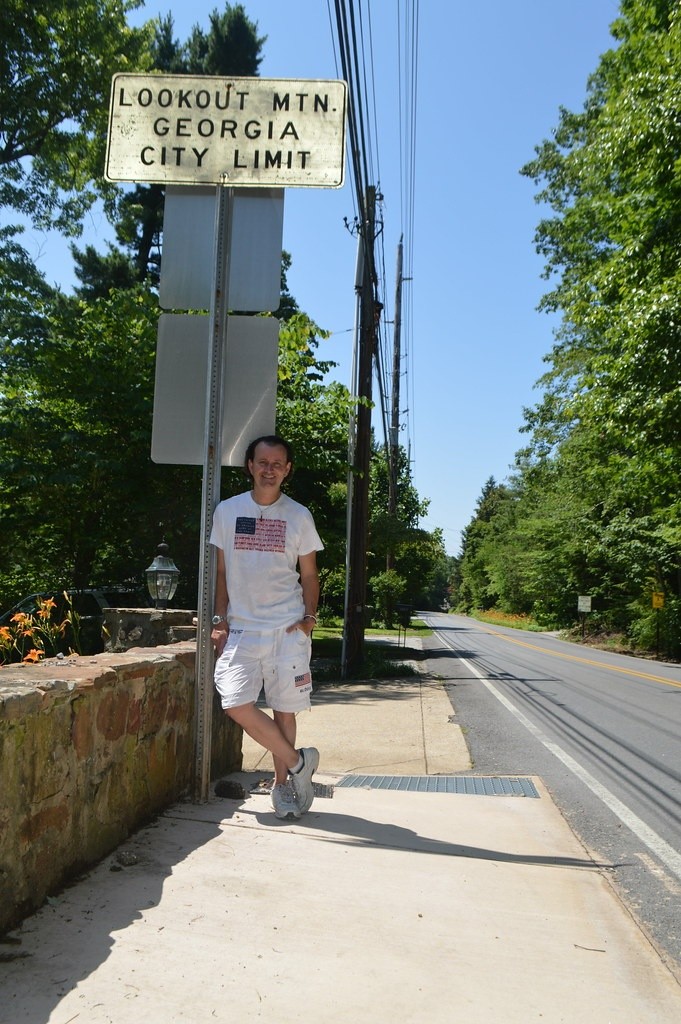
208, 436, 325, 819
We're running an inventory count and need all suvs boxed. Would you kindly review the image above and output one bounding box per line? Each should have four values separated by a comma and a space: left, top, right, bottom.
0, 583, 154, 663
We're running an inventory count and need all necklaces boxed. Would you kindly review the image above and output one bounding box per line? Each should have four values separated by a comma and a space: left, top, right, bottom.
251, 492, 279, 522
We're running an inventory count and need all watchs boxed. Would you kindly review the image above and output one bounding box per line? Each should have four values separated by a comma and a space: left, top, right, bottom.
212, 615, 226, 624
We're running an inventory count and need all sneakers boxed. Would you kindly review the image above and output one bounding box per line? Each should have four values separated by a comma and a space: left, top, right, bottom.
288, 747, 320, 814
270, 784, 301, 821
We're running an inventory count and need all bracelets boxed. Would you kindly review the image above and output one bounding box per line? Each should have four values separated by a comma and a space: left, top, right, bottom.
304, 614, 317, 623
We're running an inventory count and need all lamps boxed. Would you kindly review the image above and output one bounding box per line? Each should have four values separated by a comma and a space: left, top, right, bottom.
144, 541, 181, 611
